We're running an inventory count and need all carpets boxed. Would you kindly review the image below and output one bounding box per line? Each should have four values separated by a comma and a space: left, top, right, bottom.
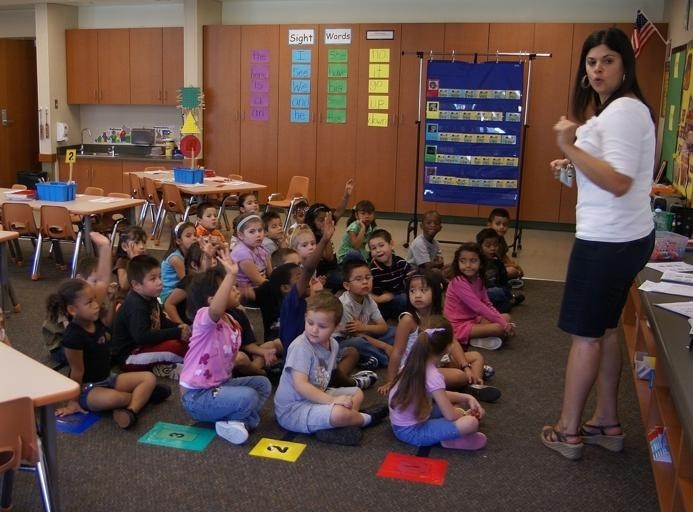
0, 251, 659, 512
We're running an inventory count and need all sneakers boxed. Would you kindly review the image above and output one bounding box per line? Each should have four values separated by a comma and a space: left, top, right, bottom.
482, 364, 495, 378
511, 292, 526, 305
441, 430, 488, 451
469, 336, 504, 350
361, 356, 379, 371
351, 368, 378, 388
359, 400, 391, 423
149, 383, 172, 402
111, 407, 136, 429
465, 382, 502, 401
214, 419, 249, 444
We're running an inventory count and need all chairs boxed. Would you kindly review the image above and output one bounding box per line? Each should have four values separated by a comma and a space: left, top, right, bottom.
155, 183, 190, 249
128, 173, 154, 227
2, 203, 50, 273
77, 184, 105, 256
95, 193, 135, 261
140, 177, 166, 240
31, 204, 83, 284
264, 176, 310, 234
0, 397, 56, 511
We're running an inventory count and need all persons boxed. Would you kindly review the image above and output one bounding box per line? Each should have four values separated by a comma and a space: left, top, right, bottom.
197, 236, 277, 377
110, 254, 191, 382
230, 212, 272, 308
487, 208, 524, 280
289, 196, 309, 234
230, 193, 258, 250
540, 27, 656, 461
238, 248, 302, 344
388, 315, 488, 451
304, 179, 356, 261
442, 241, 515, 351
375, 268, 502, 403
41, 256, 115, 368
160, 222, 197, 301
268, 210, 378, 389
406, 211, 450, 290
287, 223, 327, 291
274, 291, 389, 446
368, 229, 412, 318
476, 228, 525, 313
46, 231, 172, 430
195, 202, 228, 249
111, 226, 147, 312
261, 211, 290, 256
179, 246, 271, 445
331, 260, 396, 371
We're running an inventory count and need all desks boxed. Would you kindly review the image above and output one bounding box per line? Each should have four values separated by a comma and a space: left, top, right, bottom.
1, 222, 22, 324
129, 170, 268, 233
0, 187, 143, 267
0, 340, 81, 511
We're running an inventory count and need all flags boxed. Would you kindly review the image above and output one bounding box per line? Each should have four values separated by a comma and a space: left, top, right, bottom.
629, 8, 659, 58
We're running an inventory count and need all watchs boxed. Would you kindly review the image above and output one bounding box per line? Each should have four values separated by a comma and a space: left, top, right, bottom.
461, 363, 471, 370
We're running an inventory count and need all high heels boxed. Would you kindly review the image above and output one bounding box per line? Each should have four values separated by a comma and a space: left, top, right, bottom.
540, 426, 584, 460
580, 421, 628, 454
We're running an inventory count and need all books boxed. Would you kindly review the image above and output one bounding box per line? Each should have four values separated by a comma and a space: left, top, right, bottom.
647, 425, 672, 463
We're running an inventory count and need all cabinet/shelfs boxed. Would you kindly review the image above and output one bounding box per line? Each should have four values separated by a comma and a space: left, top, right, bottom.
359, 19, 445, 221
278, 20, 359, 218
123, 152, 185, 199
616, 21, 672, 164
130, 26, 185, 106
203, 25, 279, 204
622, 244, 693, 512
64, 28, 130, 105
435, 19, 535, 225
519, 16, 616, 230
59, 154, 122, 198
